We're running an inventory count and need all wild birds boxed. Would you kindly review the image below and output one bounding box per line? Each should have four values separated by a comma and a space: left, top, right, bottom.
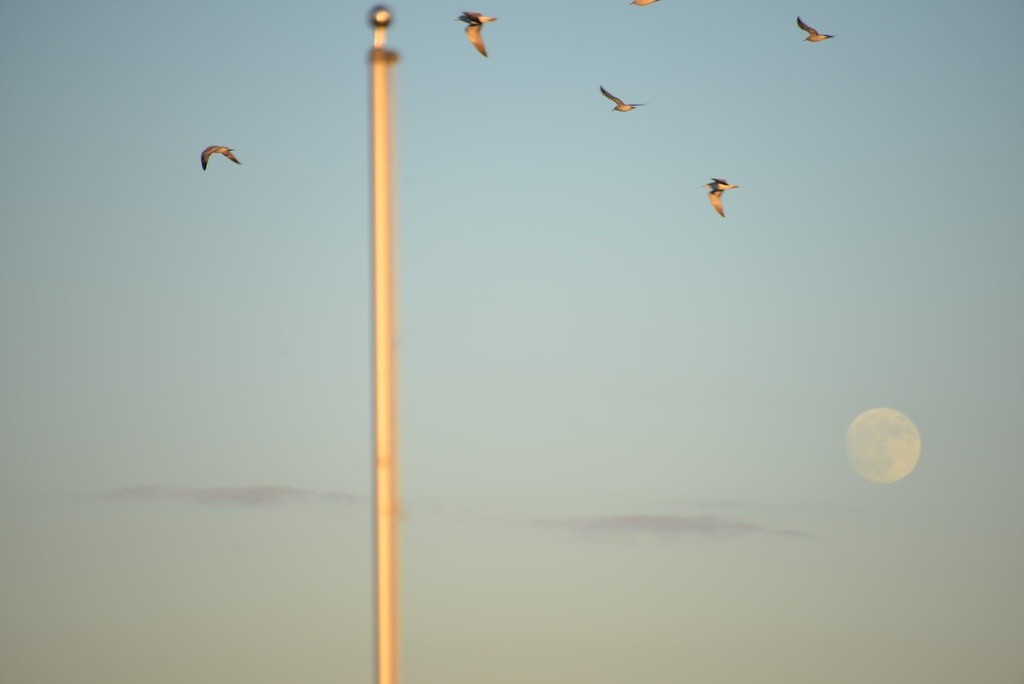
629, 0, 657, 7
201, 145, 241, 171
454, 11, 496, 58
702, 177, 739, 218
796, 16, 835, 43
599, 85, 643, 112
